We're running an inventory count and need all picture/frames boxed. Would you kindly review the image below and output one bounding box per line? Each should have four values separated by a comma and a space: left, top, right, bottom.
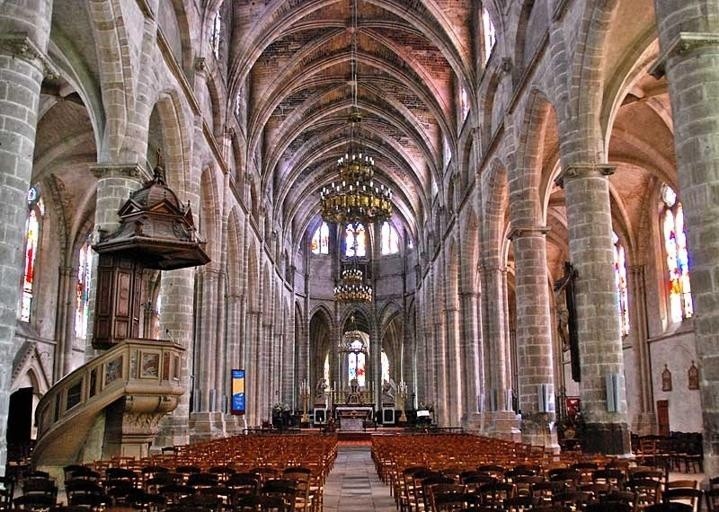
230, 369, 247, 416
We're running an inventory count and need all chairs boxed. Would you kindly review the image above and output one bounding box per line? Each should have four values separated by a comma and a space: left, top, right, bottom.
369, 427, 718, 512
0, 425, 339, 511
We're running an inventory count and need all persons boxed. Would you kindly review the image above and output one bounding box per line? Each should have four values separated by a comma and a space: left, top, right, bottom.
515, 410, 522, 432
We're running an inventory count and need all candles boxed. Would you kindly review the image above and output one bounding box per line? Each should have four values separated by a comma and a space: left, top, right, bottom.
299, 379, 310, 396
333, 381, 336, 391
397, 378, 409, 397
368, 381, 374, 392
